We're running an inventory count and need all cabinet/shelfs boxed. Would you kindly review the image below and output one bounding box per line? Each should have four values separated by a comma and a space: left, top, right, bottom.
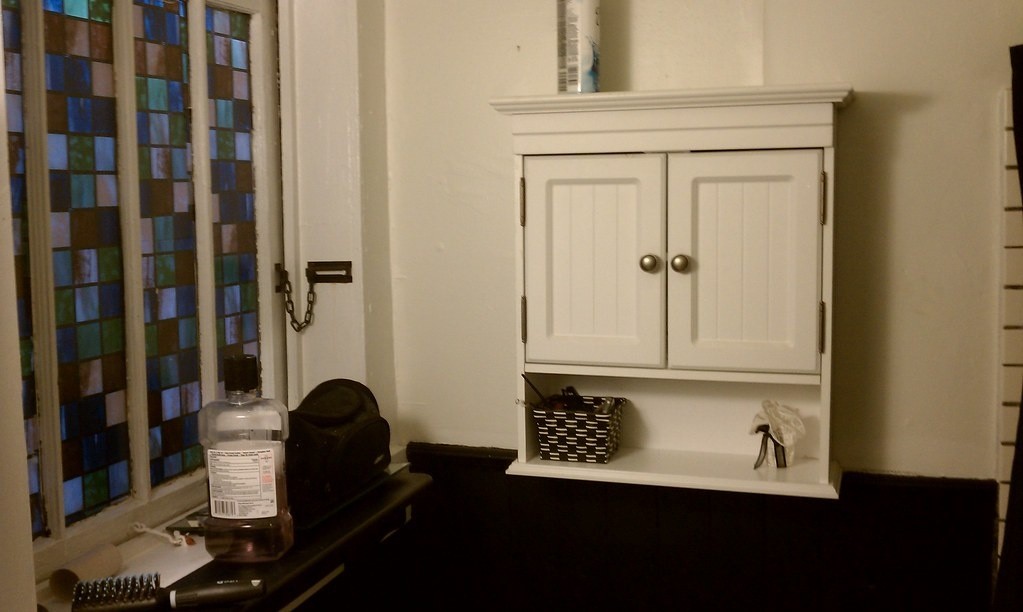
490, 81, 857, 500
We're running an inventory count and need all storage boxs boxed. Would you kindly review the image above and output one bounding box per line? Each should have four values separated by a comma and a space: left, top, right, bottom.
535, 392, 627, 465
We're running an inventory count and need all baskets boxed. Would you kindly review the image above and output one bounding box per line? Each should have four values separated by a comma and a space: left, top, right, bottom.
533, 394, 629, 464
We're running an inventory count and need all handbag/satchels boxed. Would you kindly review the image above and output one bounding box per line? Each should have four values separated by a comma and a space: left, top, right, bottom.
286, 379, 392, 525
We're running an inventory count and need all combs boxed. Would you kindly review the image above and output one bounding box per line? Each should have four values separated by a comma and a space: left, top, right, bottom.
72, 572, 264, 612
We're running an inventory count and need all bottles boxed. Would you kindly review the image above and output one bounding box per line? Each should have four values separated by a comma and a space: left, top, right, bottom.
557, 0, 600, 93
197, 353, 296, 563
